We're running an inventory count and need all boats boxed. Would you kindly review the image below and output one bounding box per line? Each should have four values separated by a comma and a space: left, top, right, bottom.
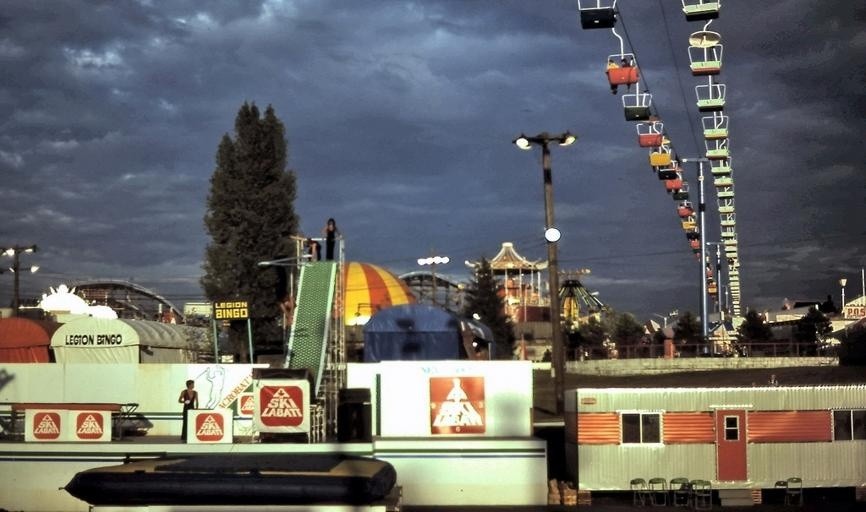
62, 451, 398, 508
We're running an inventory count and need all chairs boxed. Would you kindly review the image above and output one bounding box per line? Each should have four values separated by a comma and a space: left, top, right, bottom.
630, 477, 803, 510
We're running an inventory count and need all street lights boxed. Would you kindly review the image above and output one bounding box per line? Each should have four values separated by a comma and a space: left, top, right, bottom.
513, 129, 579, 415
417, 248, 450, 307
0, 243, 39, 316
650, 312, 678, 328
255, 253, 311, 270
839, 274, 848, 319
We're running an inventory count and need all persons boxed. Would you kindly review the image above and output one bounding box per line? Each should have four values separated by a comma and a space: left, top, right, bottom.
608, 60, 620, 95
179, 380, 198, 440
323, 217, 341, 260
304, 236, 322, 260
621, 58, 630, 91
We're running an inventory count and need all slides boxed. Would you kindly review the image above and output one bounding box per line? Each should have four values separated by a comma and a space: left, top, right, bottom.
284, 260, 338, 399
